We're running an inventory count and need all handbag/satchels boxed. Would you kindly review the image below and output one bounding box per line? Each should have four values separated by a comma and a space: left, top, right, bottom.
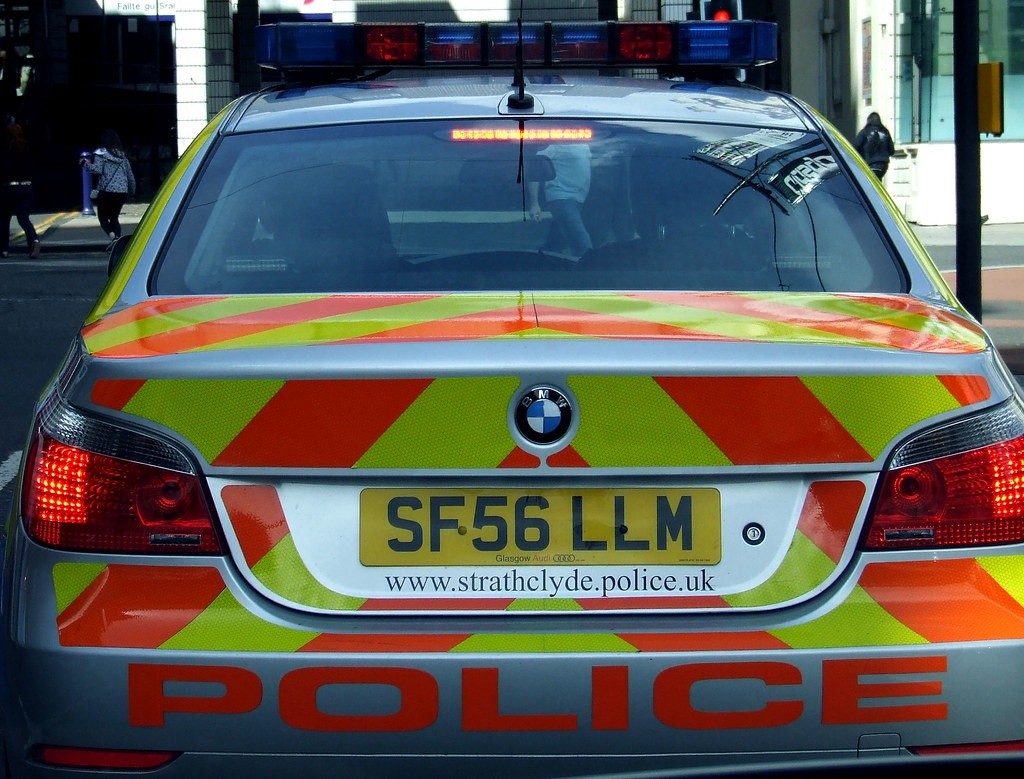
89, 189, 104, 203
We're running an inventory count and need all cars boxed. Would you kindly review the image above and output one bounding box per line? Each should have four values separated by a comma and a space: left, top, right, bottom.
0, 10, 1024, 779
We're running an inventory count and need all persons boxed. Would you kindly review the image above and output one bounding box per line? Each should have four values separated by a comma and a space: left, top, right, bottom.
529, 144, 593, 256
0, 124, 40, 259
79, 129, 136, 251
852, 112, 895, 182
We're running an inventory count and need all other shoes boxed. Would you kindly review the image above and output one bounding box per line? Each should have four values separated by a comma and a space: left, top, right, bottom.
0, 251, 8, 258
106, 238, 118, 251
29, 239, 39, 258
